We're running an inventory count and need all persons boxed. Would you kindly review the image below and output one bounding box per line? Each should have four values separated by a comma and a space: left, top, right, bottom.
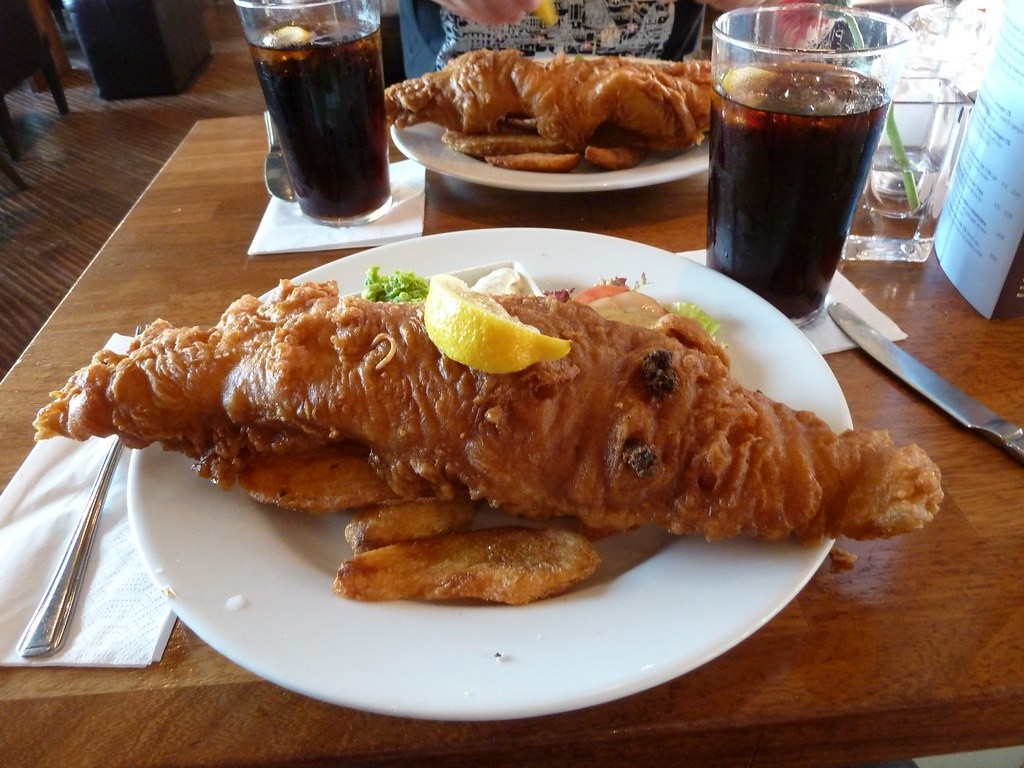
434, 1, 764, 74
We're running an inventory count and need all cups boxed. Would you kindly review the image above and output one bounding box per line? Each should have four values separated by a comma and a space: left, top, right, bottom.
706, 4, 914, 330
842, 77, 973, 262
236, 1, 391, 227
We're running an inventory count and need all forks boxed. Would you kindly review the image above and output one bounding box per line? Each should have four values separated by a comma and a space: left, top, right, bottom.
17, 323, 146, 656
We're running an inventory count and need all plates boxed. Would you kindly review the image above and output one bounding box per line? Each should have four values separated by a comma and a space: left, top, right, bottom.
130, 228, 854, 722
391, 56, 710, 193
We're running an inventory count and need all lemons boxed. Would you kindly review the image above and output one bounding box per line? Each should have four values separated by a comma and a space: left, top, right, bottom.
423, 273, 573, 374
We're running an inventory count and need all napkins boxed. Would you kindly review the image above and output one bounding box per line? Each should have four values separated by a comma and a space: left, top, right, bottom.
675, 247, 908, 356
0, 330, 181, 669
248, 158, 427, 255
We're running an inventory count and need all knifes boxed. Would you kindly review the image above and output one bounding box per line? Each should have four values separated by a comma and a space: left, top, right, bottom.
262, 111, 296, 202
828, 303, 1024, 455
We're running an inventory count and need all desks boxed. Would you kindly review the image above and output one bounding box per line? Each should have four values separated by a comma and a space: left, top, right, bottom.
0, 115, 1024, 768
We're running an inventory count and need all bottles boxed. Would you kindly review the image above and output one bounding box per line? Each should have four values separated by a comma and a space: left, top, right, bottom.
863, 0, 984, 219
